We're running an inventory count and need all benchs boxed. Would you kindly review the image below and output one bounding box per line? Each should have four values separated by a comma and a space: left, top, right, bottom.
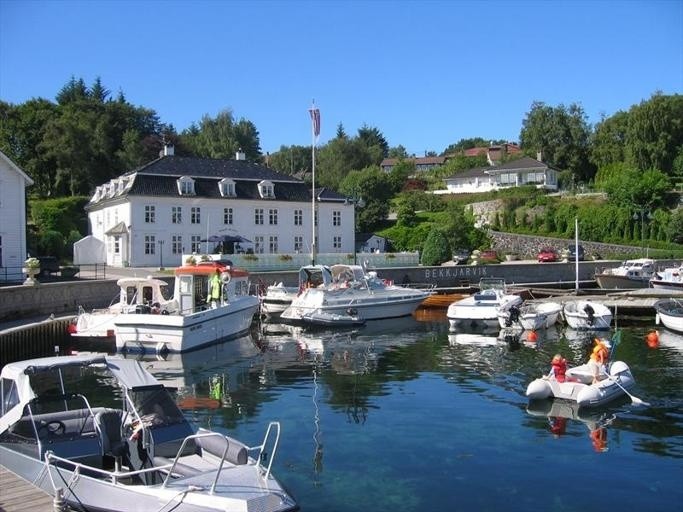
8, 406, 122, 451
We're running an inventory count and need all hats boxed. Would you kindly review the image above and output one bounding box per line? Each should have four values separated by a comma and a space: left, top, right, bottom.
604, 340, 613, 348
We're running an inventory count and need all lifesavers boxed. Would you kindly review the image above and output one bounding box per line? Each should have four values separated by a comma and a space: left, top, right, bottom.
220, 271, 231, 284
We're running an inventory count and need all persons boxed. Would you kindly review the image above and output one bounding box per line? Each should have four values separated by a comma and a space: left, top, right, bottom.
543, 354, 583, 384
205, 271, 229, 311
527, 329, 538, 342
591, 337, 609, 384
647, 328, 659, 348
150, 302, 162, 314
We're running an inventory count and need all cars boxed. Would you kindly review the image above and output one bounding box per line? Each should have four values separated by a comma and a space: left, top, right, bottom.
537, 244, 585, 263
452, 244, 498, 265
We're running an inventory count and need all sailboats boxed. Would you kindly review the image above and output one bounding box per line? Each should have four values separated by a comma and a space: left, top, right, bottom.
280, 98, 438, 321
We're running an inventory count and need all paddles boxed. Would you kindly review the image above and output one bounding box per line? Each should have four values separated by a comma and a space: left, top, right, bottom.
595, 361, 643, 404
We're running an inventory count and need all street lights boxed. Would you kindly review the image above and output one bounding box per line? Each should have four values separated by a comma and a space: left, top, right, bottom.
158, 240, 165, 267
633, 206, 654, 257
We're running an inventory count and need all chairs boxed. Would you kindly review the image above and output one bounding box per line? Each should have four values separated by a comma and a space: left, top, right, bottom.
94, 411, 132, 474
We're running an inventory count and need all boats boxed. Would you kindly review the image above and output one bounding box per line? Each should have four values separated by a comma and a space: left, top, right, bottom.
592, 258, 660, 290
300, 306, 367, 328
447, 276, 524, 320
497, 301, 562, 331
421, 286, 534, 307
524, 390, 636, 453
251, 279, 298, 313
525, 338, 637, 409
563, 299, 613, 330
67, 273, 169, 339
1, 345, 297, 512
653, 298, 683, 334
113, 214, 263, 353
648, 261, 683, 290
